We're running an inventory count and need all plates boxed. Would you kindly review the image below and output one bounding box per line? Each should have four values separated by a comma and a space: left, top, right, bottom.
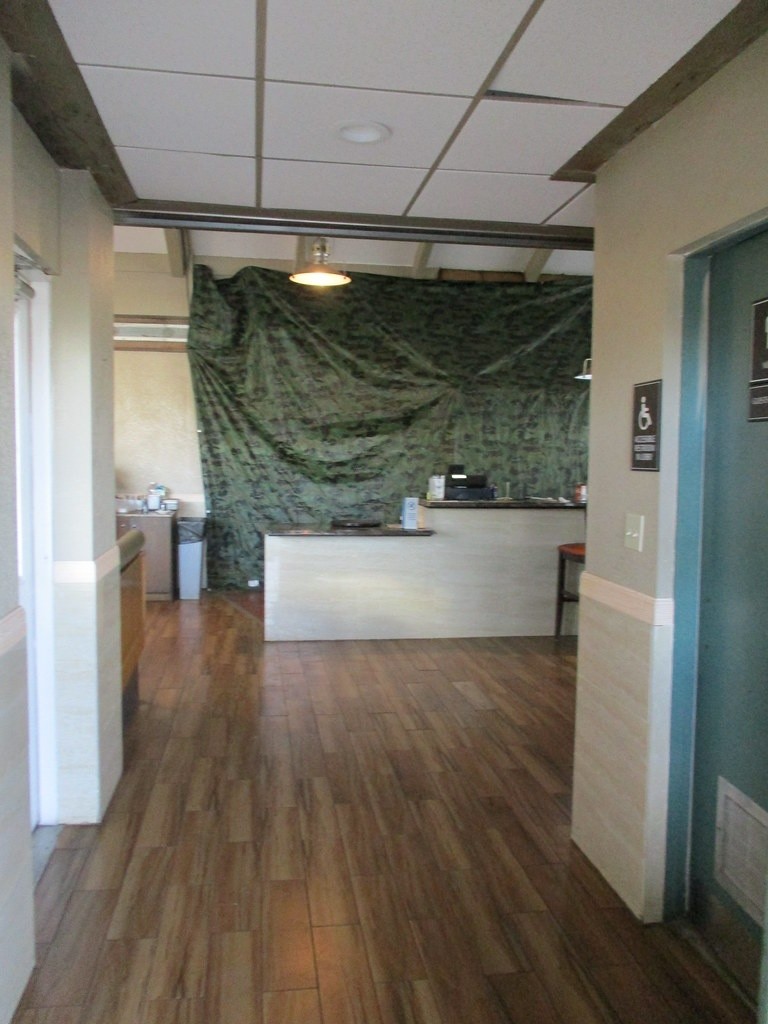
155, 510, 171, 515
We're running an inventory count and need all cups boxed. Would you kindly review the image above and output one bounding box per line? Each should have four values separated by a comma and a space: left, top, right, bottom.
117, 496, 142, 513
147, 495, 160, 509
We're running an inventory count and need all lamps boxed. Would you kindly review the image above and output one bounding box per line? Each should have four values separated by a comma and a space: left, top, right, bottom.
289, 236, 351, 286
573, 358, 593, 380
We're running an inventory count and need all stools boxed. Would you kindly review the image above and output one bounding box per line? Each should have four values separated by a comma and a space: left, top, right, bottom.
555, 542, 585, 640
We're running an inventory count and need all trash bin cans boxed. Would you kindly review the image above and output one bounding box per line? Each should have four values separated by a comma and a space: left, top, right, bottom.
176, 516, 207, 600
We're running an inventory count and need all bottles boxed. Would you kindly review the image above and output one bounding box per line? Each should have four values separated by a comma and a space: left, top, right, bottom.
580, 484, 586, 502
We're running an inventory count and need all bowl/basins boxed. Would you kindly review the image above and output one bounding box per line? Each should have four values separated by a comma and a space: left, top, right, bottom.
163, 500, 179, 510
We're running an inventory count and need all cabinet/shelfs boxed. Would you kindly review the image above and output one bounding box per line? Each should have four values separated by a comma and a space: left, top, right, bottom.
116, 528, 145, 693
116, 508, 176, 602
